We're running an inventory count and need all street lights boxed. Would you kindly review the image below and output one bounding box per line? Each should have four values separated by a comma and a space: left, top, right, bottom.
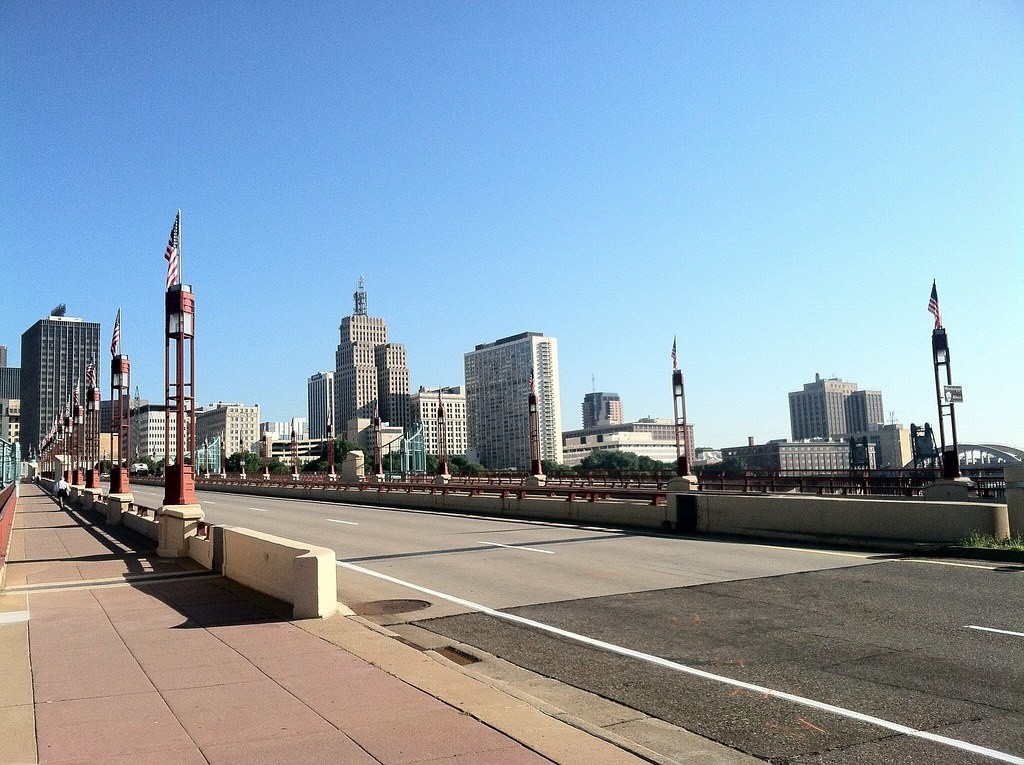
931, 328, 961, 478
71, 405, 84, 485
84, 387, 100, 489
205, 444, 210, 474
528, 393, 543, 475
220, 441, 226, 474
326, 424, 335, 475
263, 436, 270, 474
107, 354, 130, 492
239, 439, 246, 474
437, 408, 449, 475
374, 417, 383, 474
290, 431, 300, 474
36, 415, 73, 483
672, 369, 691, 476
163, 283, 197, 507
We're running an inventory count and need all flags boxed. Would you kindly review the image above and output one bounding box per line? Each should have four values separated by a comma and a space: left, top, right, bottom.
163, 213, 179, 288
87, 360, 96, 389
111, 310, 120, 357
74, 385, 80, 404
42, 402, 70, 447
927, 283, 943, 330
528, 368, 535, 393
671, 336, 677, 369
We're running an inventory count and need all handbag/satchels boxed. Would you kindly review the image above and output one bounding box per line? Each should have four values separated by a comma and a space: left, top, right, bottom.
57, 489, 67, 497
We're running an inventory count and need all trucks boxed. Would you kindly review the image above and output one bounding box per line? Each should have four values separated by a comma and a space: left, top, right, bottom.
130, 463, 149, 477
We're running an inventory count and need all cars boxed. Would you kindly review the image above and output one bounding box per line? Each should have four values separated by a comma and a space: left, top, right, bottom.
101, 473, 110, 479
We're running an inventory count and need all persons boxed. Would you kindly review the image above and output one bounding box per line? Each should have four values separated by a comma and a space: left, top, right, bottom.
54, 476, 70, 511
